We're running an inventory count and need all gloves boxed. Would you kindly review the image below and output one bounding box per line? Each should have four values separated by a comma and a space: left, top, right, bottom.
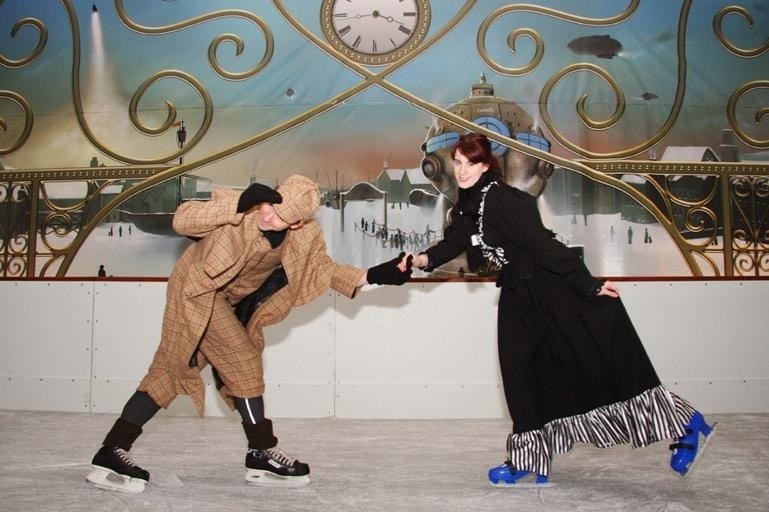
367, 252, 414, 285
237, 183, 283, 213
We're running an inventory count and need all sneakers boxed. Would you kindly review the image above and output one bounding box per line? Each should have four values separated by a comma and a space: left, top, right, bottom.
245, 447, 309, 475
92, 445, 150, 481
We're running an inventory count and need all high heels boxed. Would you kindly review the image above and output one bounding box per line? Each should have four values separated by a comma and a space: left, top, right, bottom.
488, 458, 548, 484
670, 409, 712, 474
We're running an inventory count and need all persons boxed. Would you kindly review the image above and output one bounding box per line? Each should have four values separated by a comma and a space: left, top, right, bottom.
91, 173, 414, 480
571, 214, 656, 244
397, 132, 712, 483
358, 199, 436, 251
96, 221, 134, 277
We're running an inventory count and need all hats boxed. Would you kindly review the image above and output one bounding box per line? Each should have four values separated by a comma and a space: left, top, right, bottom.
272, 174, 322, 225
